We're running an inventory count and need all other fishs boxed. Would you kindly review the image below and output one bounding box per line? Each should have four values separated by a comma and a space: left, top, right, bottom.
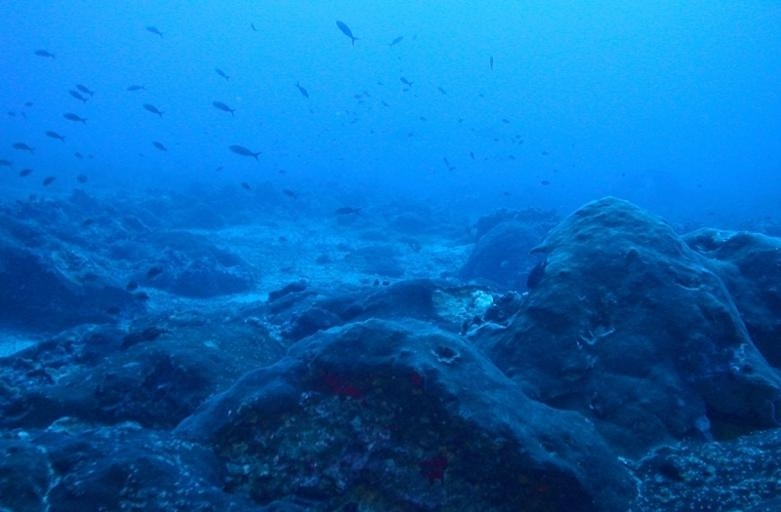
0, 19, 552, 347
